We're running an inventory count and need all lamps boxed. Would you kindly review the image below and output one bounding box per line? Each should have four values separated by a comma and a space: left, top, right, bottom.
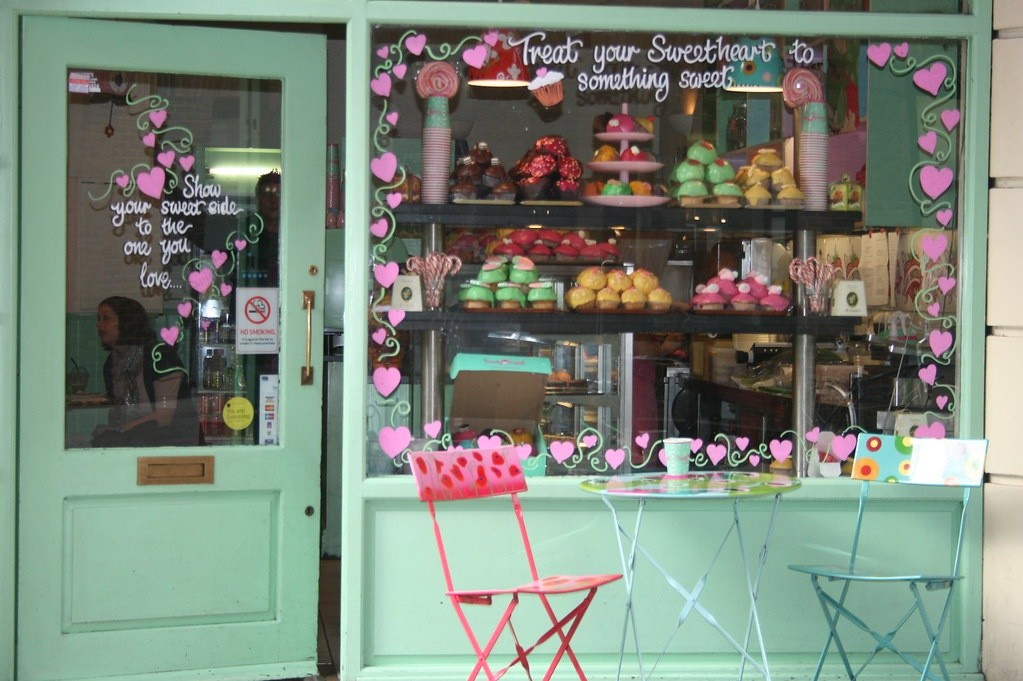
722, 0, 786, 92
467, 0, 532, 87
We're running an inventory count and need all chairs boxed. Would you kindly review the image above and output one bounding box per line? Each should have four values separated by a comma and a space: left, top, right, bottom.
787, 433, 989, 681
406, 445, 623, 681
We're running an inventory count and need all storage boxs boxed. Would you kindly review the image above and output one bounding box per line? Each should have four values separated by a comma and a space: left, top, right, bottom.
449, 353, 553, 476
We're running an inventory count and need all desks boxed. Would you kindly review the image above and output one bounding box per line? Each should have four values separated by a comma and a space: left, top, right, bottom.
65, 394, 112, 410
576, 471, 802, 681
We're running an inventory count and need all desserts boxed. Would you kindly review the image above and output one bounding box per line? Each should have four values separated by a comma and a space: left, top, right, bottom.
383, 116, 806, 313
450, 424, 537, 466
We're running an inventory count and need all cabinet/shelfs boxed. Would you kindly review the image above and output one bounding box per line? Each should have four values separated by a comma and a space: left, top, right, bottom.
371, 201, 873, 472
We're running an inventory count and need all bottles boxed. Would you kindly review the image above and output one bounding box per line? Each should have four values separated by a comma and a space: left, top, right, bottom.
450, 172, 480, 200
662, 261, 695, 312
492, 177, 518, 200
456, 157, 482, 185
202, 347, 236, 391
469, 142, 493, 168
482, 157, 507, 186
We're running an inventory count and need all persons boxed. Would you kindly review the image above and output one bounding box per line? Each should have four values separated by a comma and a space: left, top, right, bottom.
89, 297, 208, 446
634, 242, 740, 468
233, 168, 281, 444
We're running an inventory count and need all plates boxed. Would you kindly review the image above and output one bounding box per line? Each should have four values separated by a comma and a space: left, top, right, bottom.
595, 133, 654, 142
732, 332, 777, 353
587, 161, 664, 172
580, 195, 670, 207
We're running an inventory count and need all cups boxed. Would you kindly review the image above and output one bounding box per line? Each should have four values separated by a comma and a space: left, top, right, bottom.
802, 280, 833, 316
421, 94, 452, 205
421, 274, 447, 314
799, 102, 829, 211
326, 144, 340, 210
664, 438, 692, 474
819, 463, 841, 477
